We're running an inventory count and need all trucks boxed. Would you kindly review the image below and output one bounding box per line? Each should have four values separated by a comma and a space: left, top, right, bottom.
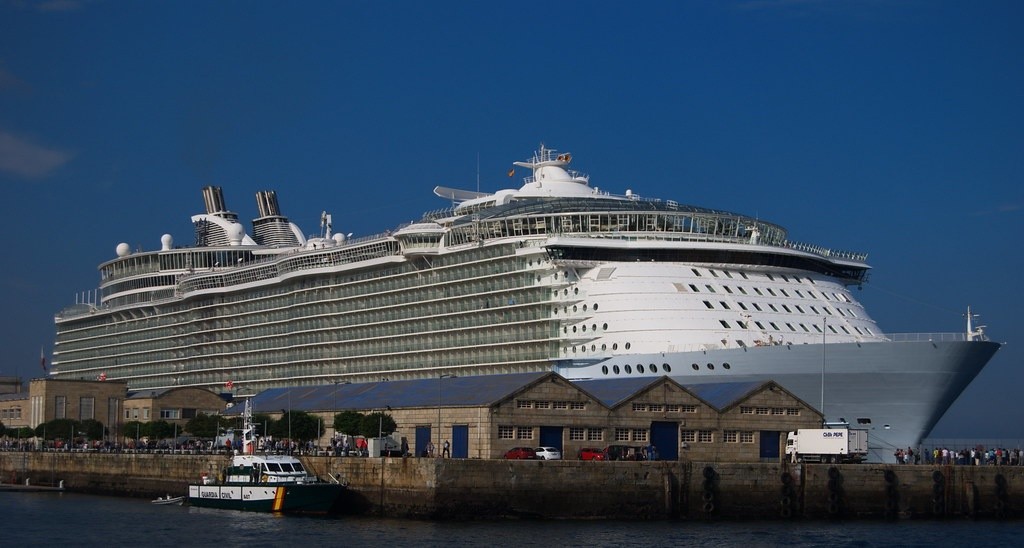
785, 429, 869, 464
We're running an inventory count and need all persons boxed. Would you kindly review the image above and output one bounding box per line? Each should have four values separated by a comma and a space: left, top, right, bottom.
602, 443, 657, 461
791, 447, 798, 462
0, 436, 452, 457
893, 446, 1024, 466
250, 467, 260, 483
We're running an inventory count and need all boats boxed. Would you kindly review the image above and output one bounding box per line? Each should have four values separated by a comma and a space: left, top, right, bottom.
0, 479, 66, 492
193, 454, 347, 518
151, 495, 189, 506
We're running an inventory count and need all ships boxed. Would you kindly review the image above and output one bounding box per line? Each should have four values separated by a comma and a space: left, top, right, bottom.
45, 144, 1006, 462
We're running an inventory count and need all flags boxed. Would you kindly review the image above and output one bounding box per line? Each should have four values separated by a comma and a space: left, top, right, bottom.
227, 380, 234, 391
41, 349, 46, 370
98, 373, 107, 381
509, 169, 514, 177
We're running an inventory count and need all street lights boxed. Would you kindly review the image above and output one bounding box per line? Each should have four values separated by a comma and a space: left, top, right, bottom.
235, 387, 253, 428
331, 380, 351, 450
437, 373, 457, 459
378, 404, 391, 458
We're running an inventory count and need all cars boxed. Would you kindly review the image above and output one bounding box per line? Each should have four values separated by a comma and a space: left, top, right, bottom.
577, 447, 604, 461
533, 447, 561, 461
504, 446, 536, 460
